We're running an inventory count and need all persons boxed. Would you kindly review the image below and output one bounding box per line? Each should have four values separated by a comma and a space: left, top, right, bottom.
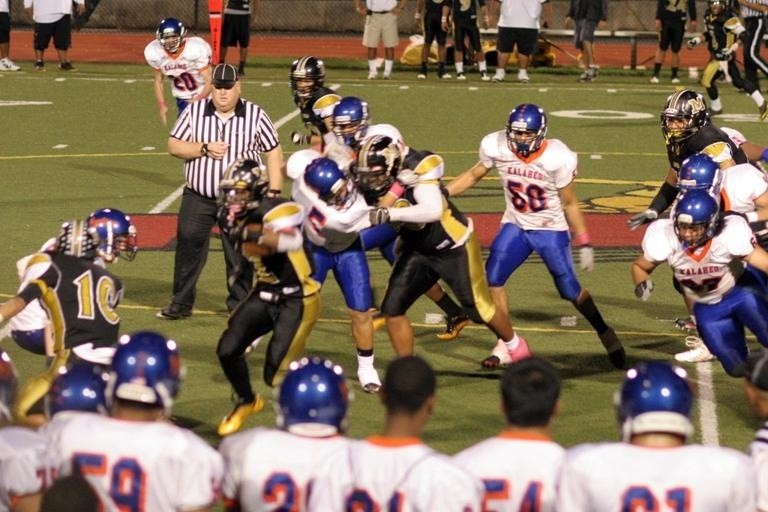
1, 329, 226, 512
0, 218, 123, 421
454, 356, 568, 512
23, 1, 86, 72
649, 0, 767, 81
565, 349, 768, 512
218, 0, 260, 73
624, 81, 768, 375
143, 17, 214, 126
357, 0, 607, 85
10, 204, 141, 358
165, 56, 637, 436
686, 0, 768, 119
217, 353, 488, 512
0, 1, 19, 75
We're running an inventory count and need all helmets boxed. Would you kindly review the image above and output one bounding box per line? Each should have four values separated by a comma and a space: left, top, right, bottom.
354, 136, 401, 195
304, 158, 351, 203
332, 97, 369, 144
289, 56, 324, 98
221, 159, 268, 206
674, 154, 721, 249
45, 333, 181, 410
279, 358, 350, 437
59, 208, 139, 264
505, 104, 547, 156
661, 91, 708, 147
156, 18, 188, 53
619, 363, 694, 436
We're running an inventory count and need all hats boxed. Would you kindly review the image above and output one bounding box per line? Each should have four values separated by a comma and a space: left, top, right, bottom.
210, 63, 238, 86
732, 353, 768, 393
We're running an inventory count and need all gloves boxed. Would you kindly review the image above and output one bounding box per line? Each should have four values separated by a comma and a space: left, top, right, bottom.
289, 131, 310, 148
580, 243, 595, 273
722, 45, 738, 55
688, 37, 701, 49
370, 207, 387, 227
628, 208, 657, 230
635, 279, 653, 302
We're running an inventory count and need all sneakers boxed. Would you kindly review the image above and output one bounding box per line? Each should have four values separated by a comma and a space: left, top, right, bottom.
59, 61, 78, 71
356, 355, 382, 394
671, 79, 681, 83
217, 393, 265, 435
759, 100, 768, 121
437, 312, 474, 338
482, 340, 532, 368
706, 106, 723, 116
366, 70, 392, 80
33, 60, 43, 68
598, 328, 626, 368
417, 67, 528, 82
650, 77, 659, 83
576, 65, 597, 82
676, 317, 700, 337
162, 302, 192, 319
1, 58, 21, 72
675, 336, 715, 362
